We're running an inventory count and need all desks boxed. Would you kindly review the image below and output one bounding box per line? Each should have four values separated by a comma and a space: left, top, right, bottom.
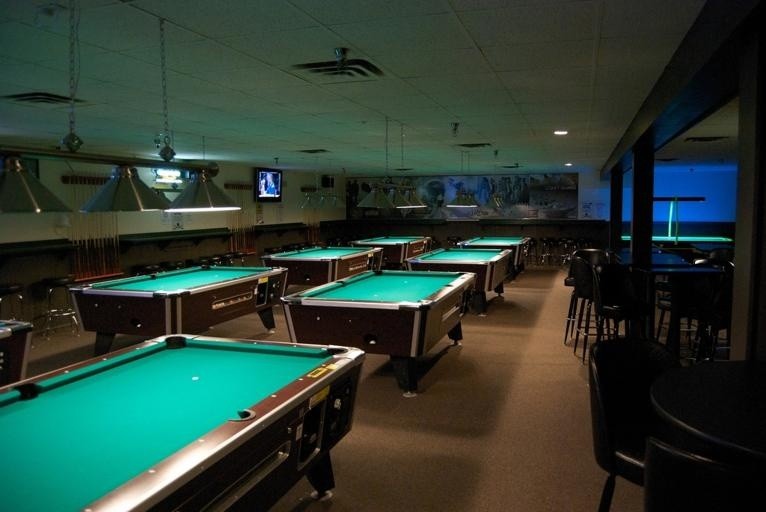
350, 236, 432, 269
403, 248, 513, 317
0, 320, 35, 387
280, 270, 476, 398
0, 333, 366, 512
261, 246, 385, 294
457, 235, 531, 283
69, 265, 290, 358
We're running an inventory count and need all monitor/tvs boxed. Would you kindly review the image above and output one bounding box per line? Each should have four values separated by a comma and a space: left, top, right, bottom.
253, 167, 282, 203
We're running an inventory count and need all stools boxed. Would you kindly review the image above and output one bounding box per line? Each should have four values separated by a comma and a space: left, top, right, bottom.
1, 284, 35, 348
132, 241, 307, 275
37, 274, 82, 340
432, 235, 573, 270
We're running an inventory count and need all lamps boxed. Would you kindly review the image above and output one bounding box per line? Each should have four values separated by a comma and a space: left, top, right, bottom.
76, 161, 172, 212
445, 150, 481, 207
163, 162, 241, 212
486, 150, 505, 209
2, 154, 75, 212
357, 116, 429, 210
300, 154, 347, 209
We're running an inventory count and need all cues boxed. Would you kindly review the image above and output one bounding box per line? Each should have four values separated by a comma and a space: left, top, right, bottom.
67, 167, 120, 282
227, 183, 256, 258
305, 183, 323, 244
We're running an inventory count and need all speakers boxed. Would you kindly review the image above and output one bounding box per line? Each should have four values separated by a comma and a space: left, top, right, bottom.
321, 175, 334, 187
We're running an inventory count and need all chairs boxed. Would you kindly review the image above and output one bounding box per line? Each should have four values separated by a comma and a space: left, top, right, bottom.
565, 247, 766, 512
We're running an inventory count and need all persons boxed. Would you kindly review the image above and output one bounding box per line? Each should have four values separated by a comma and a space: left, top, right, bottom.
261, 173, 275, 195
